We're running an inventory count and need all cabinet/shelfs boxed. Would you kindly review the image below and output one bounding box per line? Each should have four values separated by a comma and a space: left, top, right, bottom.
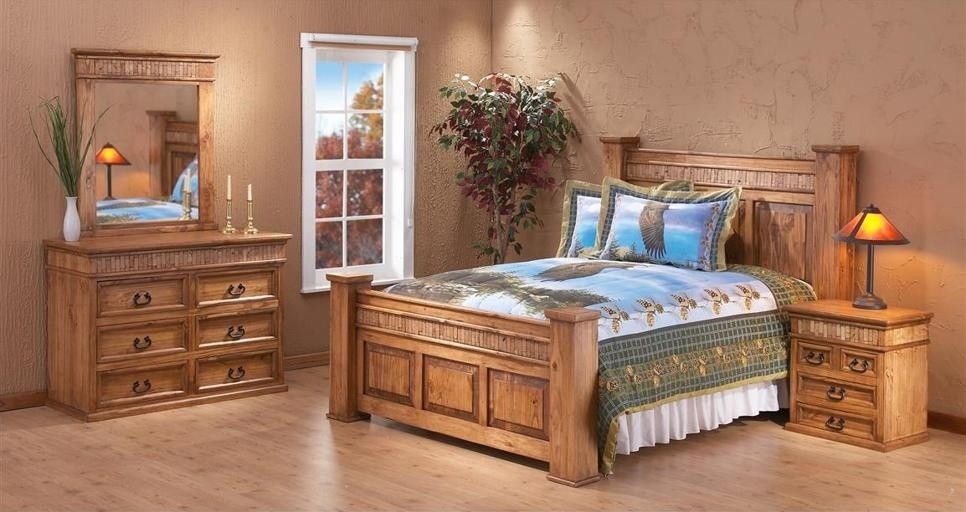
42, 229, 293, 422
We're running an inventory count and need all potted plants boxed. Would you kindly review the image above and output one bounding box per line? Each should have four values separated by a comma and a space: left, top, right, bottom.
26, 95, 113, 243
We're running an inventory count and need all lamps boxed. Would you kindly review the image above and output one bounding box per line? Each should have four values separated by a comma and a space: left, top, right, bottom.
95, 142, 131, 200
831, 204, 911, 310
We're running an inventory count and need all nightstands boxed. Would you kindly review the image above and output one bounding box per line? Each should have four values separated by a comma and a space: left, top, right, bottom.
782, 299, 934, 454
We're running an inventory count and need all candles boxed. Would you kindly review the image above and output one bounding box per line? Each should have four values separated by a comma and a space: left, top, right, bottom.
227, 174, 232, 200
247, 184, 252, 201
184, 169, 192, 193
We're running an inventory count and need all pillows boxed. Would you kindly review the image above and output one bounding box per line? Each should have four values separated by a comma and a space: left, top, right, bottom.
168, 154, 199, 207
555, 178, 694, 259
590, 175, 743, 272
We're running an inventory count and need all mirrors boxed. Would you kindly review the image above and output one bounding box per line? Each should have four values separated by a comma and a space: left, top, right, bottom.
70, 48, 222, 238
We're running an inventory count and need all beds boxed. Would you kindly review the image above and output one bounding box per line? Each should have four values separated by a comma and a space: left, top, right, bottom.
326, 137, 860, 487
96, 109, 199, 222
